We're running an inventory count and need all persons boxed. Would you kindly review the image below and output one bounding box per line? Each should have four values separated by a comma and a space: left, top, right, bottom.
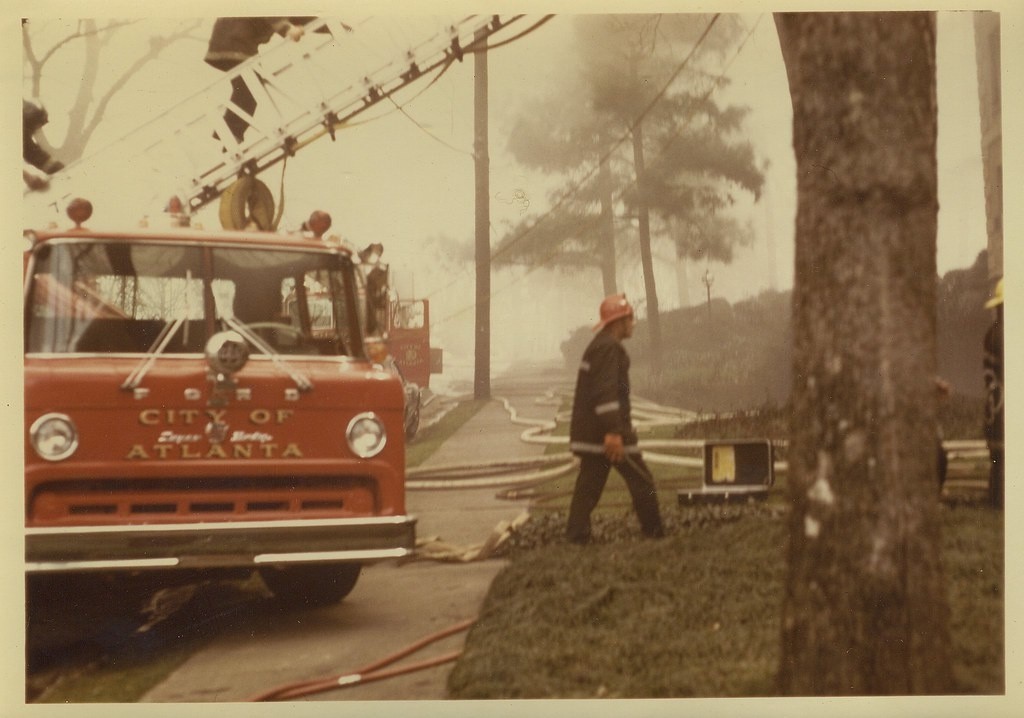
566, 295, 675, 547
984, 277, 1005, 510
935, 378, 950, 492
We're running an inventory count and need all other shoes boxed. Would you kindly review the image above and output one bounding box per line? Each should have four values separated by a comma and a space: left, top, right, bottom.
643, 531, 669, 541
565, 535, 596, 546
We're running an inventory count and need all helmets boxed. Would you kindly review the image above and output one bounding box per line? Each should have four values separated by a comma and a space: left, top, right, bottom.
592, 295, 633, 332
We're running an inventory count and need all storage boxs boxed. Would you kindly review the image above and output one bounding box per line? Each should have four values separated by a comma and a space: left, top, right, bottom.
675, 437, 776, 506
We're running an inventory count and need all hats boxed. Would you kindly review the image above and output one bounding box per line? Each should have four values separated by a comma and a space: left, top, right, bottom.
984, 277, 1004, 310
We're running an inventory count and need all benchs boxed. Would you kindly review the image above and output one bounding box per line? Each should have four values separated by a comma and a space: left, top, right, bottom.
27, 316, 227, 353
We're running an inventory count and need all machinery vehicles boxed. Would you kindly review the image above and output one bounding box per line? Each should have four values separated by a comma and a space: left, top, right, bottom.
284, 288, 432, 391
19, 199, 419, 607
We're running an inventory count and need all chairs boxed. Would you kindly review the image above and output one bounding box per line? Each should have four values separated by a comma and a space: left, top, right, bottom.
232, 275, 282, 353
